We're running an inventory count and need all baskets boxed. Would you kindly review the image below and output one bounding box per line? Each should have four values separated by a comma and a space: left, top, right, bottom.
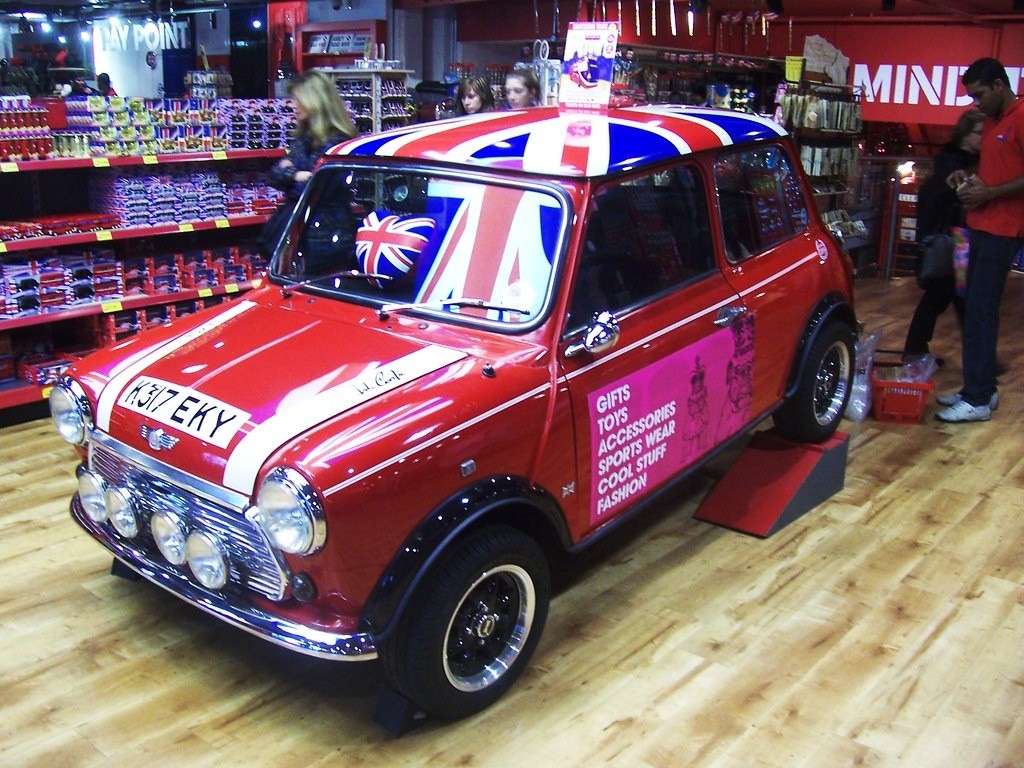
868, 365, 935, 424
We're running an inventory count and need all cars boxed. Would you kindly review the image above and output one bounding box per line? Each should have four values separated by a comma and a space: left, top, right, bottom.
46, 105, 860, 716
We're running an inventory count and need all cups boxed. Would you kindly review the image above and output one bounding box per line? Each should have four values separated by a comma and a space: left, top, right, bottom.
356, 59, 402, 69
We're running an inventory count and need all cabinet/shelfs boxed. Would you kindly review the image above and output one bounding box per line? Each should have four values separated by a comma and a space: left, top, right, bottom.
776, 78, 873, 251
316, 68, 416, 134
0, 147, 291, 413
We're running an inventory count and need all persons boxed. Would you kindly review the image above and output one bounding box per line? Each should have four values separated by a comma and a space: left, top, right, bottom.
506, 70, 541, 111
70, 74, 98, 96
269, 72, 360, 275
455, 75, 496, 117
84, 73, 118, 96
903, 109, 1011, 376
935, 58, 1024, 421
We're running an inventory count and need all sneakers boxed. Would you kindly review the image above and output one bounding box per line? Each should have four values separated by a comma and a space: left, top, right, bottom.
934, 393, 998, 409
935, 401, 991, 422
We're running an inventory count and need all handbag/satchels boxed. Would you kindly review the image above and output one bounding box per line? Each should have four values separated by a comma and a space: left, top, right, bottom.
842, 332, 879, 421
254, 198, 341, 276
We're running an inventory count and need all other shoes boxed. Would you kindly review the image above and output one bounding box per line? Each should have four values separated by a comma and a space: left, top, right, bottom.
902, 356, 945, 368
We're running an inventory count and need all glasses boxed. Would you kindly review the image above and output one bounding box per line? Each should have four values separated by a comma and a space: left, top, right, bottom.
970, 130, 983, 137
